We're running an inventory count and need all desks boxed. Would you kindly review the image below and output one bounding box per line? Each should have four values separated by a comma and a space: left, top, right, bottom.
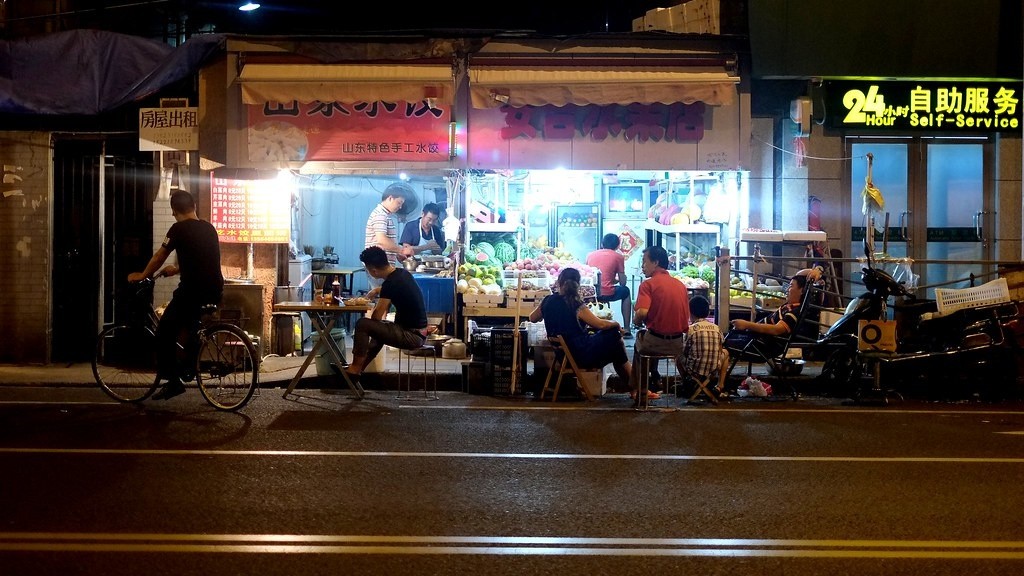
311, 266, 365, 334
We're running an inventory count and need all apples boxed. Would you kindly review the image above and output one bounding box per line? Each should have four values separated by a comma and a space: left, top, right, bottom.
505, 253, 560, 277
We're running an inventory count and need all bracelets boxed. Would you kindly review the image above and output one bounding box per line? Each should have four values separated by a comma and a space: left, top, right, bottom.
172, 265, 178, 274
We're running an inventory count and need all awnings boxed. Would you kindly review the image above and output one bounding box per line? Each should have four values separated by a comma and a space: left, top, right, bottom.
234, 61, 454, 107
467, 64, 741, 108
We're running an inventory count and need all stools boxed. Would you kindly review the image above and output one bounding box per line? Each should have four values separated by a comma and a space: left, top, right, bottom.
850, 351, 904, 407
633, 353, 677, 412
395, 345, 438, 401
688, 373, 719, 405
216, 342, 261, 397
271, 312, 304, 357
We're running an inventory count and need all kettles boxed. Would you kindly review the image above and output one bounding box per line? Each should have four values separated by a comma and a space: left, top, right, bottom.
442, 337, 467, 359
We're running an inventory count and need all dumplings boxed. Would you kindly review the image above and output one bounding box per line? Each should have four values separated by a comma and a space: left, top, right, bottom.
248, 123, 308, 160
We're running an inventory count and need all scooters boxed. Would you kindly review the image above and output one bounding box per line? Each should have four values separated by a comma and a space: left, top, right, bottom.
816, 247, 1024, 397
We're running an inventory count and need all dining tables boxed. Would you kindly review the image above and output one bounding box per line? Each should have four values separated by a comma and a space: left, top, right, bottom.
274, 300, 374, 398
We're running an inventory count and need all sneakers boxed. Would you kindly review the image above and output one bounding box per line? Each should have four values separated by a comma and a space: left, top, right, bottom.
649, 379, 664, 394
671, 380, 694, 394
151, 381, 184, 401
623, 333, 633, 339
712, 385, 729, 399
330, 362, 365, 383
630, 388, 660, 398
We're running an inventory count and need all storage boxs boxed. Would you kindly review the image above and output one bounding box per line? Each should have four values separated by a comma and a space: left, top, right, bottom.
934, 274, 1010, 311
461, 361, 526, 396
274, 285, 305, 304
412, 275, 456, 315
473, 328, 530, 363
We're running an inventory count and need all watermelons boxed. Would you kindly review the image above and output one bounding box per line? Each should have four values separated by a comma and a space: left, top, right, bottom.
467, 234, 518, 269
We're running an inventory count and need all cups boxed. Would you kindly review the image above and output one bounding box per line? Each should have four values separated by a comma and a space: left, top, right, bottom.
315, 288, 323, 304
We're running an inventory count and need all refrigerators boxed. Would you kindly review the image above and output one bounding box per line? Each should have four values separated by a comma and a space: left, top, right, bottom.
288, 253, 312, 342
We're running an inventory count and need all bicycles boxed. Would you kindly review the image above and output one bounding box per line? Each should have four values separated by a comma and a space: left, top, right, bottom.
90, 268, 264, 411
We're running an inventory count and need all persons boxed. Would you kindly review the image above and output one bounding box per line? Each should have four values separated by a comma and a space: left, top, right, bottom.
630, 246, 690, 394
328, 245, 429, 382
399, 203, 444, 258
128, 189, 224, 402
585, 233, 633, 339
529, 267, 660, 400
670, 275, 809, 398
364, 185, 415, 314
675, 295, 730, 398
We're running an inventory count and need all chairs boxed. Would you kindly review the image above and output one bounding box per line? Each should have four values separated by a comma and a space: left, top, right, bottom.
722, 277, 814, 401
541, 335, 601, 401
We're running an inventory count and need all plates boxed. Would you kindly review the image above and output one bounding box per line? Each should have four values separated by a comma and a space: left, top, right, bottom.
343, 300, 372, 305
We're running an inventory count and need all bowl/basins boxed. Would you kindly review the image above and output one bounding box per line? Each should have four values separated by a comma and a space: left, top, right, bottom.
765, 359, 807, 375
403, 260, 418, 271
425, 261, 433, 267
432, 261, 444, 267
402, 334, 453, 356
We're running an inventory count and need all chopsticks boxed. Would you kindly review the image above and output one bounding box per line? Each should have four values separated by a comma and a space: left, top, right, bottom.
323, 246, 335, 253
304, 244, 316, 257
312, 275, 326, 289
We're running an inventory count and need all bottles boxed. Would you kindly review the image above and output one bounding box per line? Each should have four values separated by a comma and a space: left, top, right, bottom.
331, 254, 339, 269
332, 275, 339, 304
320, 253, 331, 268
